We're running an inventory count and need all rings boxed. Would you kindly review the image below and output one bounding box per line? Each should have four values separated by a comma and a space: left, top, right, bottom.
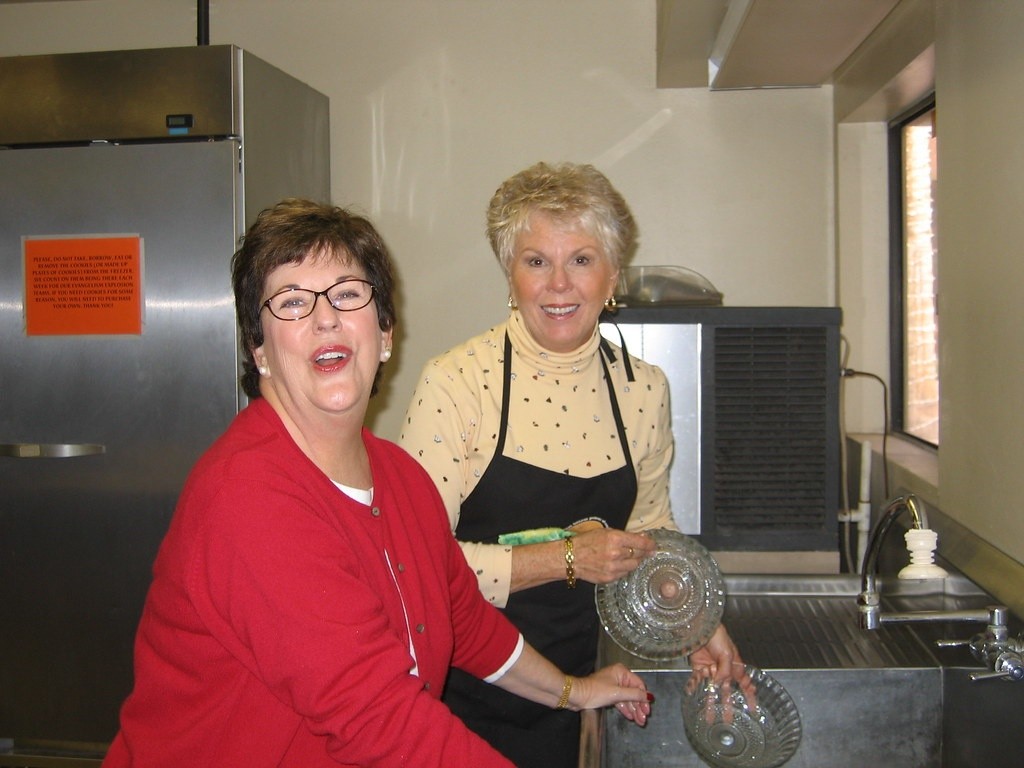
630, 548, 634, 558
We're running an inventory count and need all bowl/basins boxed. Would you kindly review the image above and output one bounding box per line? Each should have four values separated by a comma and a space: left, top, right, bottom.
595, 528, 727, 662
681, 663, 803, 768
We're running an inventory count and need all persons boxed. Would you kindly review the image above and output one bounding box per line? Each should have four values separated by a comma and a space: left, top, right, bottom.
103, 197, 654, 768
396, 163, 759, 768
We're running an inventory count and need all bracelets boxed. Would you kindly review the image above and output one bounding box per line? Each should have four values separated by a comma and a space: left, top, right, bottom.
556, 675, 572, 708
565, 535, 575, 588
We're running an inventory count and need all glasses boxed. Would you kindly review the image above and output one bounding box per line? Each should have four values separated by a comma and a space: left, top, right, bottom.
257, 278, 379, 322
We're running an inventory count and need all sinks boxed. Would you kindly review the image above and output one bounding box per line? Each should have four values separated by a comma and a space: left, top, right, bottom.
577, 664, 1023, 768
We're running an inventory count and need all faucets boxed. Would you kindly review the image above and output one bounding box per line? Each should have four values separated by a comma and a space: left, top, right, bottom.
857, 491, 948, 632
